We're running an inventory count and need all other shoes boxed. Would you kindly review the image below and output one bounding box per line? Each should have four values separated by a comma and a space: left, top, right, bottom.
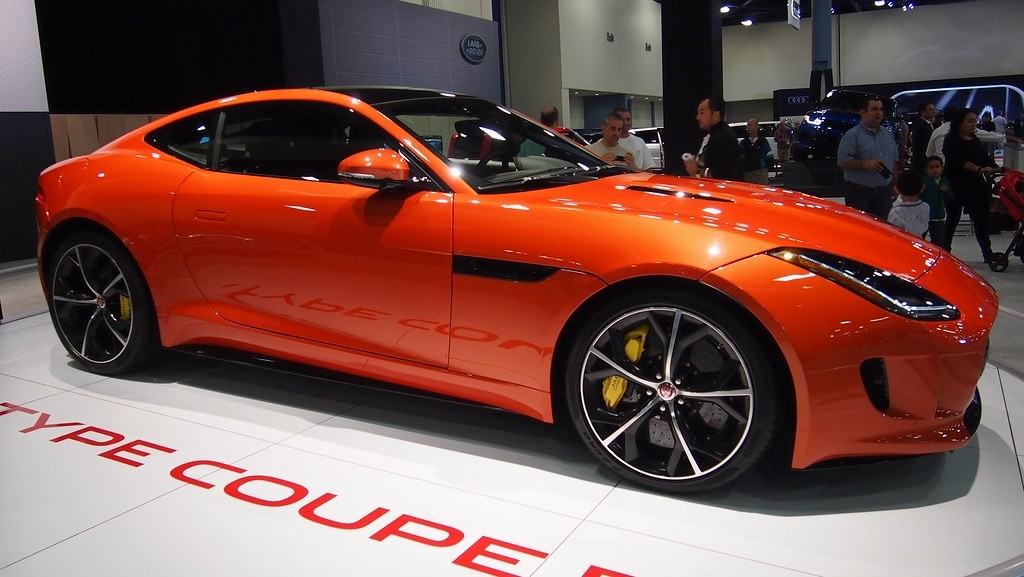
983, 253, 1003, 263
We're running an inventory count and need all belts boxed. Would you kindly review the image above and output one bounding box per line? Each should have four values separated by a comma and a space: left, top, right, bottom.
848, 184, 888, 193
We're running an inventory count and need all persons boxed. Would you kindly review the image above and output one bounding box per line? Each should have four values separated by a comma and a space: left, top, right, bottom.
514, 104, 559, 157
738, 117, 773, 189
887, 102, 1024, 264
615, 107, 657, 173
774, 119, 802, 162
681, 96, 742, 185
836, 97, 901, 223
582, 111, 641, 172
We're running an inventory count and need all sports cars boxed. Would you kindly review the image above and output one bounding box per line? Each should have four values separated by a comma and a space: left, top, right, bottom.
36, 85, 1001, 496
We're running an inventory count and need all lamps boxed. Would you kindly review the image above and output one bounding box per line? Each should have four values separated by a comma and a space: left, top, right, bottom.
739, 14, 755, 27
718, 3, 740, 14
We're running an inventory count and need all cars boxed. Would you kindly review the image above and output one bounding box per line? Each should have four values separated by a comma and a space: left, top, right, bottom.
788, 88, 913, 178
555, 126, 665, 170
727, 121, 783, 160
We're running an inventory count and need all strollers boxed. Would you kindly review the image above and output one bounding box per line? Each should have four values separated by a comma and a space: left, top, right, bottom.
979, 164, 1024, 274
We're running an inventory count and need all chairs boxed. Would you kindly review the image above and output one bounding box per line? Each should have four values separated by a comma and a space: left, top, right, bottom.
241, 126, 295, 179
347, 117, 400, 156
273, 101, 358, 184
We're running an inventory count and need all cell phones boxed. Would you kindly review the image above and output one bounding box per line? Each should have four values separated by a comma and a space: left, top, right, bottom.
877, 164, 893, 179
614, 156, 625, 162
681, 153, 693, 162
